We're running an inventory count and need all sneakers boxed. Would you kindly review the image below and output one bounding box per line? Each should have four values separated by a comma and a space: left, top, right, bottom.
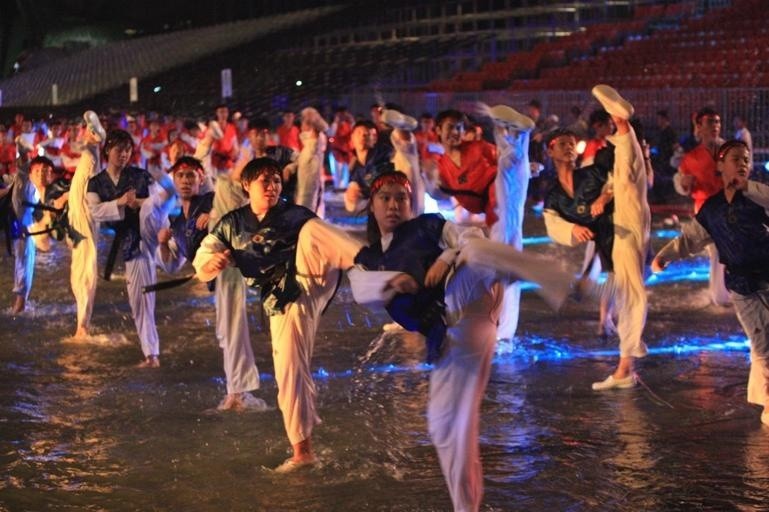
759, 408, 769, 430
488, 103, 534, 135
590, 372, 640, 394
570, 273, 618, 310
79, 110, 108, 145
272, 454, 323, 477
205, 118, 225, 144
299, 106, 330, 134
378, 109, 417, 134
592, 82, 636, 122
13, 132, 35, 154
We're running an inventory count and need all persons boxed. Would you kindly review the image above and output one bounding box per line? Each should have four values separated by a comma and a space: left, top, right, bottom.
89, 120, 222, 368
158, 153, 260, 412
655, 106, 769, 425
29, 110, 106, 342
0, 137, 36, 312
544, 86, 652, 391
193, 157, 370, 476
0, 105, 657, 258
347, 173, 605, 512
